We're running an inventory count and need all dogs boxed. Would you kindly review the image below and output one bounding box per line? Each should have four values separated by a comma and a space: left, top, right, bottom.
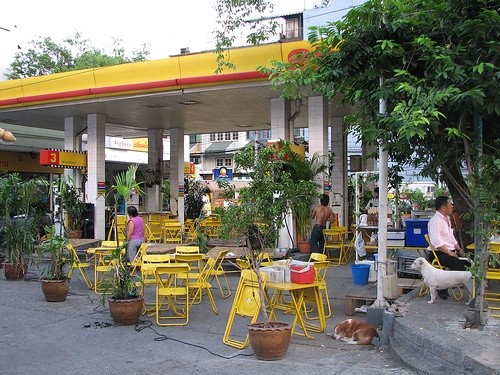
326, 318, 380, 345
409, 257, 473, 306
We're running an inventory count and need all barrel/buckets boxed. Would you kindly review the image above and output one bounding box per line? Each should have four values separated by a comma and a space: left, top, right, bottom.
351, 264, 371, 285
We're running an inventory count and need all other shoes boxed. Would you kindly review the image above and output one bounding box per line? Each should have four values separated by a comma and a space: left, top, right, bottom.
445, 288, 450, 296
438, 290, 447, 299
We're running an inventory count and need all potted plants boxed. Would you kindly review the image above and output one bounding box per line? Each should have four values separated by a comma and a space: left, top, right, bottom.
95, 241, 150, 326
37, 225, 78, 302
0, 171, 50, 281
192, 138, 337, 361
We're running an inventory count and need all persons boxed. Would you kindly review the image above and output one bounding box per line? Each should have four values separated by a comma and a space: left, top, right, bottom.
126, 206, 145, 274
364, 187, 389, 214
426, 196, 472, 300
310, 193, 334, 261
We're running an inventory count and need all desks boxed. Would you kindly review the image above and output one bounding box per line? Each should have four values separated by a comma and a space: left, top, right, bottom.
466, 241, 498, 251
142, 260, 191, 316
260, 281, 325, 339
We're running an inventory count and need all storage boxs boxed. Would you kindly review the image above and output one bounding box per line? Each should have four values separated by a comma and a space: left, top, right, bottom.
387, 232, 406, 248
290, 261, 316, 285
404, 218, 429, 248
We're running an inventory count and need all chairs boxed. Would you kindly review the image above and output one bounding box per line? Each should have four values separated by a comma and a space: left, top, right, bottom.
63, 215, 357, 348
418, 231, 464, 301
473, 243, 500, 319
321, 224, 358, 267
